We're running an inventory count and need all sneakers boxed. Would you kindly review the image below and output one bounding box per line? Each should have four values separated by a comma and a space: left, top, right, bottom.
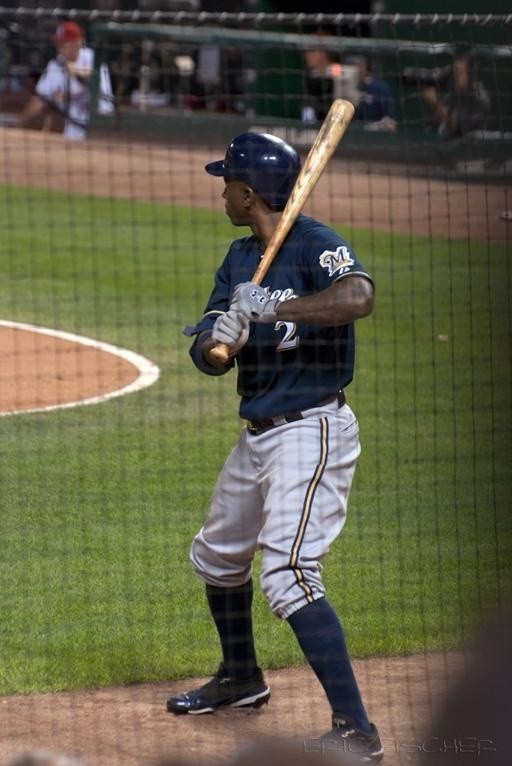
165, 661, 274, 718
303, 722, 386, 766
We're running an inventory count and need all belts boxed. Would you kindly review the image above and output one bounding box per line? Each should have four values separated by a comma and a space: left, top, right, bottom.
243, 387, 348, 437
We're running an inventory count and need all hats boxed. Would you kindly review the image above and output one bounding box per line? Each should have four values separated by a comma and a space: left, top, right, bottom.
47, 19, 85, 45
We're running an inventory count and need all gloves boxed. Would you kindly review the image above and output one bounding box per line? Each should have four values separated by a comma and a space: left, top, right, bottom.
228, 279, 281, 326
211, 310, 246, 349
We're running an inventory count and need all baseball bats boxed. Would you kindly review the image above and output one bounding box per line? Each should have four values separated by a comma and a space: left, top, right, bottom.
210, 98, 355, 365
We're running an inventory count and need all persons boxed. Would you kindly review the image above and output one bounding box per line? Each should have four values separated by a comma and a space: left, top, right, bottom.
301, 33, 490, 138
163, 133, 391, 763
106, 35, 256, 115
1, 20, 113, 140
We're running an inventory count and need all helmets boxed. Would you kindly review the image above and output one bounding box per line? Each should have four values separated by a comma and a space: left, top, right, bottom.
203, 129, 302, 213
